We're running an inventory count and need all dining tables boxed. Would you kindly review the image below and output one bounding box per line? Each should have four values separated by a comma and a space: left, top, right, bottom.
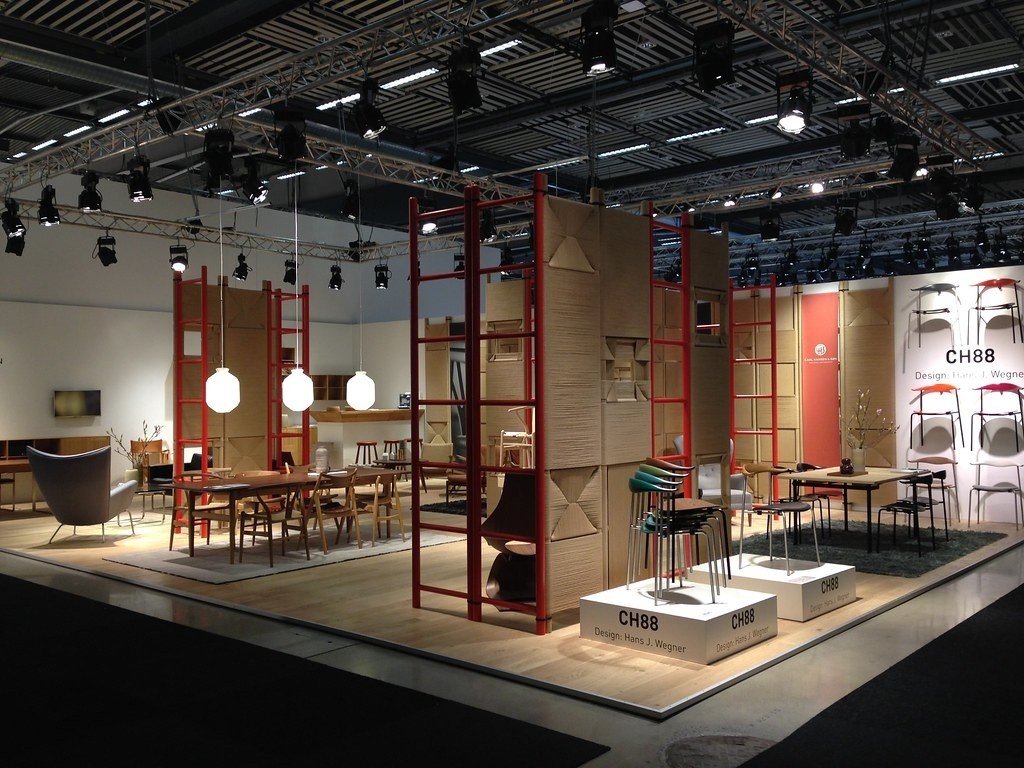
777, 465, 931, 556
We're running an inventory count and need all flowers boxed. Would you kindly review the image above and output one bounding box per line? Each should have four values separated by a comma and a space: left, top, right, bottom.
838, 388, 901, 450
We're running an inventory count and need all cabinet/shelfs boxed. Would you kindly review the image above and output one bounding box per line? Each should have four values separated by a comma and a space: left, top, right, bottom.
129, 439, 170, 484
0, 436, 111, 515
282, 425, 318, 467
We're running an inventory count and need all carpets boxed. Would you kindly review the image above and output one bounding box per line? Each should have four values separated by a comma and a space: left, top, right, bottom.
101, 516, 467, 587
732, 519, 1009, 579
409, 497, 487, 520
0, 573, 612, 768
737, 582, 1024, 768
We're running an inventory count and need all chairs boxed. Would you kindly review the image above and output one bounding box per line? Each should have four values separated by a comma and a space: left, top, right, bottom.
766, 462, 832, 546
283, 461, 340, 540
970, 383, 1024, 453
967, 278, 1023, 344
239, 481, 311, 567
167, 473, 235, 558
904, 457, 961, 527
738, 463, 821, 577
347, 472, 406, 549
967, 459, 1024, 532
295, 468, 362, 556
25, 444, 137, 545
238, 470, 290, 547
907, 283, 964, 350
876, 470, 949, 558
446, 444, 487, 506
910, 383, 965, 451
626, 457, 733, 607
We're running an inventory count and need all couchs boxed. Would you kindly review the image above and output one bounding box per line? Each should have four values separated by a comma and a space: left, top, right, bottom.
730, 473, 752, 527
148, 453, 212, 496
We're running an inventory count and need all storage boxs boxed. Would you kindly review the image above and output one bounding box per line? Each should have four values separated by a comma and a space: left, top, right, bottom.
579, 576, 779, 666
686, 553, 857, 622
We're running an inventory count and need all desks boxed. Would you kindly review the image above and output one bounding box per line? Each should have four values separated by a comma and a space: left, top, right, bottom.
165, 467, 412, 565
372, 459, 429, 497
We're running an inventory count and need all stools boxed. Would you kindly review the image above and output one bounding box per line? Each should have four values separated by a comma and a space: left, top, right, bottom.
383, 440, 400, 471
355, 441, 380, 487
402, 438, 423, 483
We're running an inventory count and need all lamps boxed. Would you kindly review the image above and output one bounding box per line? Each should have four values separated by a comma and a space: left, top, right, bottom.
662, 100, 1024, 290
282, 163, 314, 412
345, 160, 375, 411
0, 78, 537, 289
776, 67, 817, 136
206, 177, 241, 414
447, 36, 483, 117
581, 0, 618, 77
694, 18, 735, 94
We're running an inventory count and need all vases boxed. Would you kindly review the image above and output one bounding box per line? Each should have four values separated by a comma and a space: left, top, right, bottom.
132, 463, 144, 487
852, 447, 866, 473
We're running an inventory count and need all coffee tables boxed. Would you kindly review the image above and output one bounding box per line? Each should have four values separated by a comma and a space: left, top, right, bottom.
135, 487, 167, 523
